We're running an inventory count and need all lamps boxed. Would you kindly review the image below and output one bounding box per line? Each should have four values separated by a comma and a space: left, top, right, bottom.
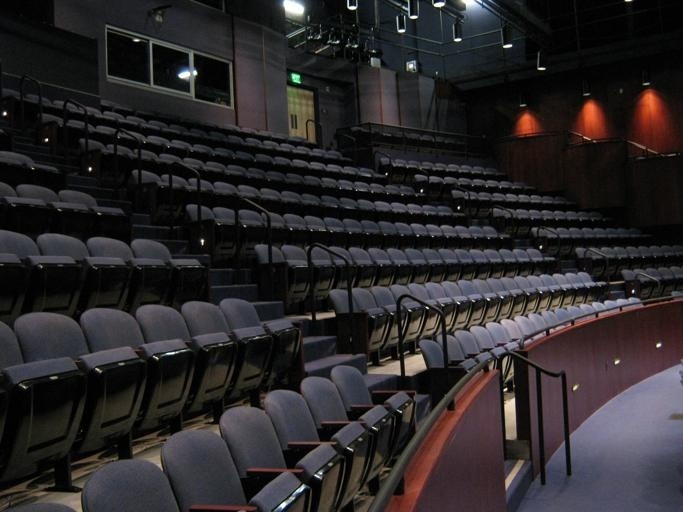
347, 0, 549, 72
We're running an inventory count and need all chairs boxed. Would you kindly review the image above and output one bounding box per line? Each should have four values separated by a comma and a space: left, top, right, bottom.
508, 177, 683, 353
1, 99, 507, 512
